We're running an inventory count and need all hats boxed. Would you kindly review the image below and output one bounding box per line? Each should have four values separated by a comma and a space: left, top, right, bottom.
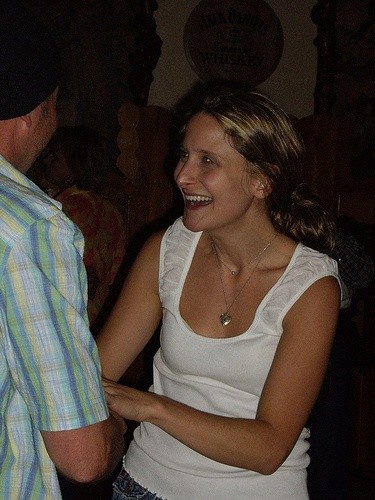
0, 0, 64, 121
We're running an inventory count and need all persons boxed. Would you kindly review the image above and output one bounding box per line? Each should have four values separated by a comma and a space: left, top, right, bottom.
40, 122, 140, 334
1, 23, 120, 500
95, 82, 351, 499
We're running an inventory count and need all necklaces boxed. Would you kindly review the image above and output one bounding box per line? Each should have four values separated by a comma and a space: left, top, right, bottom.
206, 229, 281, 328
